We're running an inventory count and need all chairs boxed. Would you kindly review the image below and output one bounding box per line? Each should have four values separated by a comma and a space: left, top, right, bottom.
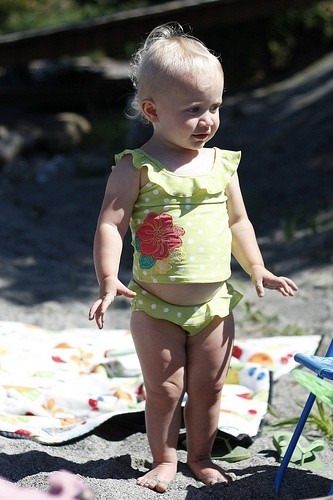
272, 338, 333, 495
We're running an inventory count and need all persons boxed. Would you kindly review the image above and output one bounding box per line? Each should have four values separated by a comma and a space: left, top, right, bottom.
88, 26, 298, 492
